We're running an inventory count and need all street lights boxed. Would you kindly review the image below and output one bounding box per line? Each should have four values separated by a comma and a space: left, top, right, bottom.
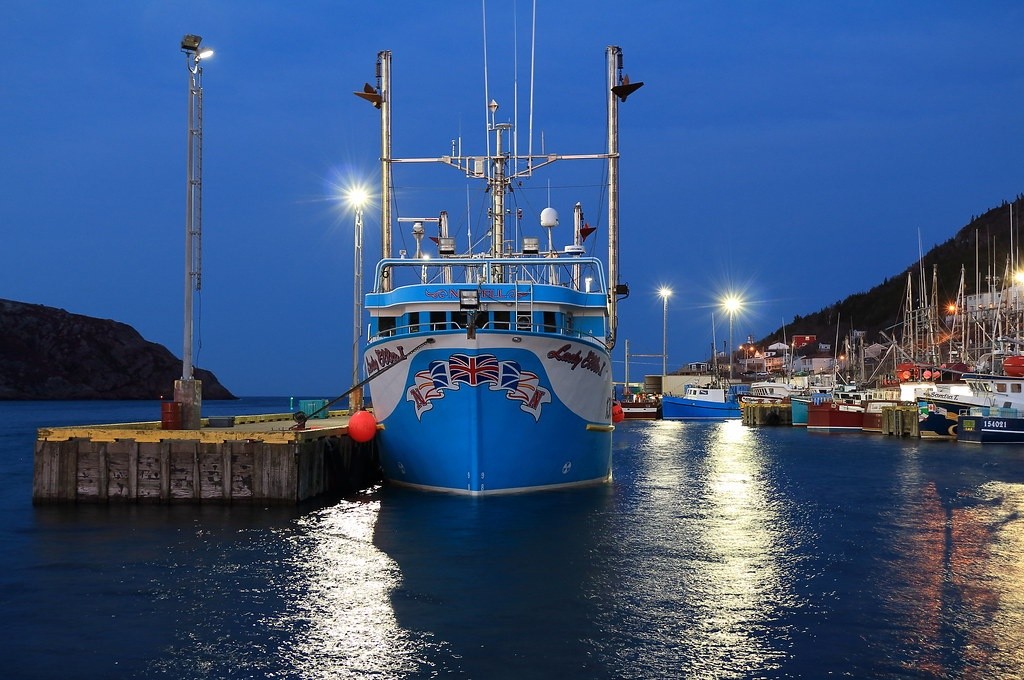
739, 346, 754, 359
349, 186, 370, 416
172, 31, 215, 430
725, 298, 739, 380
660, 287, 671, 375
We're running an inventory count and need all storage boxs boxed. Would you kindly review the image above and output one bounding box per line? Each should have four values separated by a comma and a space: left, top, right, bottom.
298, 399, 329, 419
208, 415, 235, 427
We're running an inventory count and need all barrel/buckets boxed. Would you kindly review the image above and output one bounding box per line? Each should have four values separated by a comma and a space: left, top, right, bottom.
161, 401, 182, 430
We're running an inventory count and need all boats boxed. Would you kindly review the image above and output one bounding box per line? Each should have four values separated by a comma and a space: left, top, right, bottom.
662, 312, 751, 421
363, 0, 644, 507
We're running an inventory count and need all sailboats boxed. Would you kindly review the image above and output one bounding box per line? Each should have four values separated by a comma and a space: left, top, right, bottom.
790, 203, 1024, 446
